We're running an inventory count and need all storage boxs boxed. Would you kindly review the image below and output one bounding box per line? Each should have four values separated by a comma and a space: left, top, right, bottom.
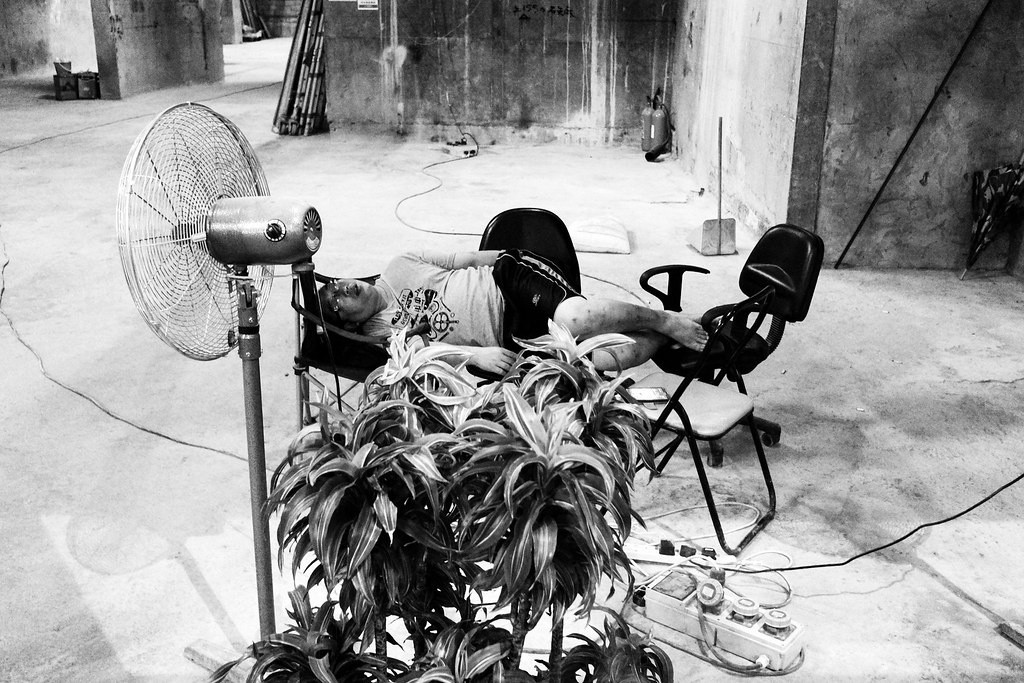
56, 90, 77, 102
53, 74, 76, 90
79, 79, 101, 99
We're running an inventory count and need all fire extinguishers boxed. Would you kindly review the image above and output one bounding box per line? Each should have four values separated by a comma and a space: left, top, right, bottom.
645, 103, 672, 162
641, 86, 676, 151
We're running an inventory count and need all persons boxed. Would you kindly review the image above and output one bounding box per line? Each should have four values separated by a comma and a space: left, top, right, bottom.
316, 248, 722, 374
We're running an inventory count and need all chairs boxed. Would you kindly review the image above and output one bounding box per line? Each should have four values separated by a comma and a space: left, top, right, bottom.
463, 207, 582, 386
621, 262, 796, 556
285, 271, 430, 433
636, 223, 830, 467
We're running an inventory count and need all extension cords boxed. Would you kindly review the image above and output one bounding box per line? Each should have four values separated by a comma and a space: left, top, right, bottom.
624, 544, 737, 571
443, 144, 477, 157
645, 572, 805, 671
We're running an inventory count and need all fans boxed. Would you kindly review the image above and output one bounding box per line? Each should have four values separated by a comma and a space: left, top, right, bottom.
114, 102, 325, 642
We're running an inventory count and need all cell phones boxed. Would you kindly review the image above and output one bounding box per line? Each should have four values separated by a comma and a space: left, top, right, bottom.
626, 387, 670, 403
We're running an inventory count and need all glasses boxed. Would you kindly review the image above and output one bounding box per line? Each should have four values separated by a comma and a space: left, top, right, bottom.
328, 278, 341, 323
